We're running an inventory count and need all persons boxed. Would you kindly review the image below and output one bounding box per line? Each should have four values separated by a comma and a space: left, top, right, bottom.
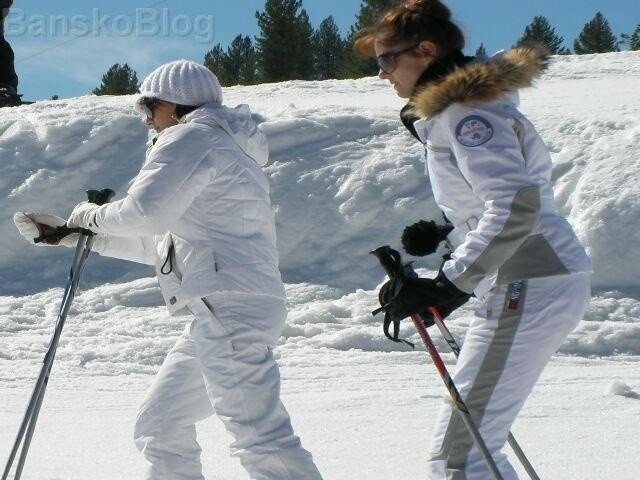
351, 1, 593, 479
14, 58, 324, 479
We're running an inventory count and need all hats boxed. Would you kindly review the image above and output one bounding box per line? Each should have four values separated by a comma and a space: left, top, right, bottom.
139, 60, 224, 107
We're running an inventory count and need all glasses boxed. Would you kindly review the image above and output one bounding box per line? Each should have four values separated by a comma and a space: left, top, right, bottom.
138, 99, 158, 120
374, 41, 422, 75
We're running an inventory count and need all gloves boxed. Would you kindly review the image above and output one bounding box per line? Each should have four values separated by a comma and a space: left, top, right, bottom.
65, 199, 100, 236
12, 208, 79, 248
378, 269, 472, 330
